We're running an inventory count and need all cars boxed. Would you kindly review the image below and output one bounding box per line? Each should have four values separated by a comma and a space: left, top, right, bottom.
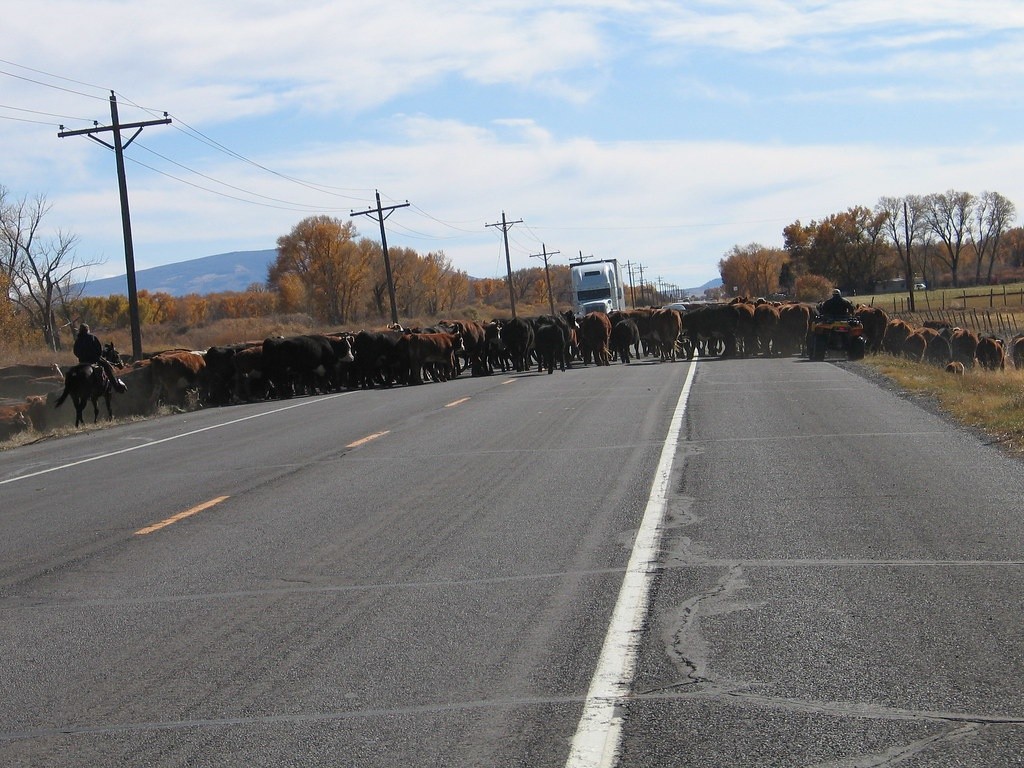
913, 284, 926, 291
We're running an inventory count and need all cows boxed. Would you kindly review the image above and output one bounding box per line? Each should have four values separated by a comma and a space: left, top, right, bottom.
0, 294, 1024, 443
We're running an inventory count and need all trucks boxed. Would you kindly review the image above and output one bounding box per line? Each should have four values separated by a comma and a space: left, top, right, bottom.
570, 258, 626, 315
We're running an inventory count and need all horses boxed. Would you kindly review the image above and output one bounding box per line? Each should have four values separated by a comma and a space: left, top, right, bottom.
52, 341, 124, 429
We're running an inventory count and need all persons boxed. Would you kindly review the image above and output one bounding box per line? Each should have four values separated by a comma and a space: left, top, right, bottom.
73, 324, 126, 392
822, 289, 855, 315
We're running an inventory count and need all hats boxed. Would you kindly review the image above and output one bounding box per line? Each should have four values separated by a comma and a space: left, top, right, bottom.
832, 289, 841, 295
